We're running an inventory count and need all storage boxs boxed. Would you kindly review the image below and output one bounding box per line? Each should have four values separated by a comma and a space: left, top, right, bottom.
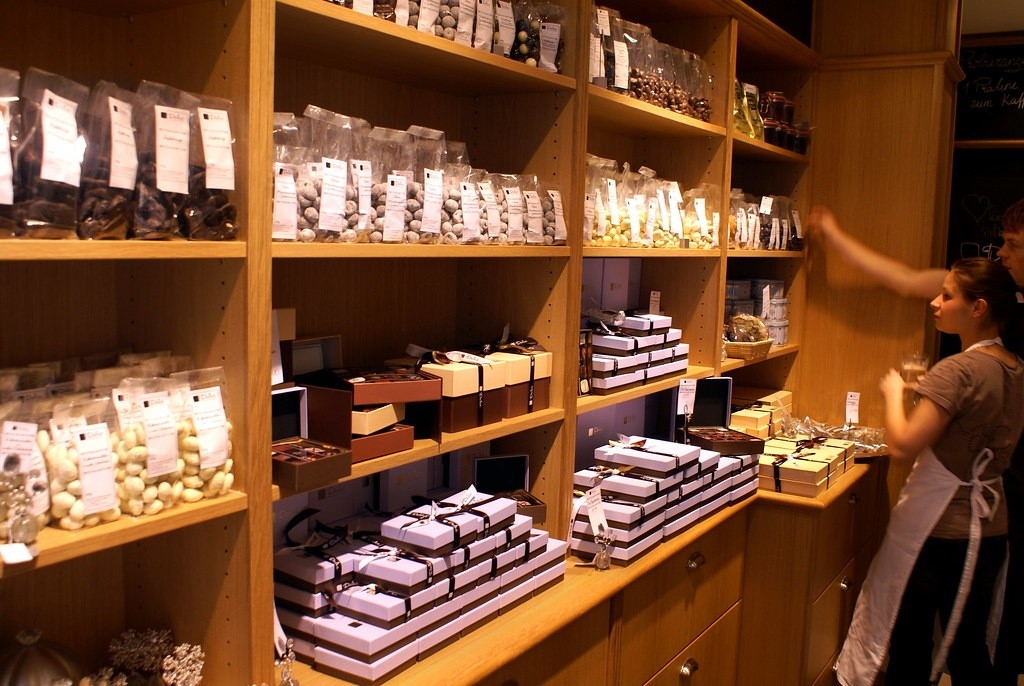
273, 322, 567, 686
570, 308, 855, 568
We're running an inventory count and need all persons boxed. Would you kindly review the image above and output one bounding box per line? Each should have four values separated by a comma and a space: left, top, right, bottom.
811, 190, 1024, 686
830, 258, 1024, 686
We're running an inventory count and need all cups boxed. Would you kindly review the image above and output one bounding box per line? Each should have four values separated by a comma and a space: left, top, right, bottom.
899, 353, 930, 401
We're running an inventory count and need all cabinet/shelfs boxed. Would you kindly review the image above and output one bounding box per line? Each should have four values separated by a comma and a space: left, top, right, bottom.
0, 0, 819, 686
608, 504, 749, 686
736, 459, 880, 686
